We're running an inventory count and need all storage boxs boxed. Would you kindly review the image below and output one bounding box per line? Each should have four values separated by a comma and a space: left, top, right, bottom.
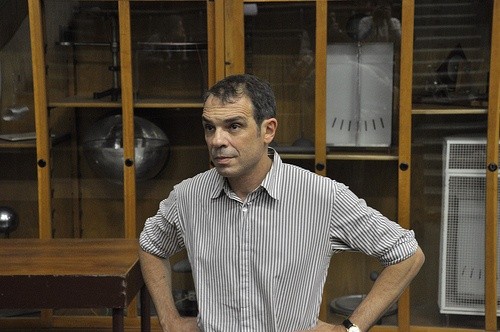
438, 138, 500, 318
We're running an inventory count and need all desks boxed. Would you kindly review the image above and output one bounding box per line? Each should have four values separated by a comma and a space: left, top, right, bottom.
0, 238, 151, 332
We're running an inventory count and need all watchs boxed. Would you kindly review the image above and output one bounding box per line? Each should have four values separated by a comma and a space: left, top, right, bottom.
342, 319, 360, 332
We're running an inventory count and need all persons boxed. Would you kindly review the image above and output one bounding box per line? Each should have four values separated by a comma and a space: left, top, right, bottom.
300, 0, 401, 83
138, 74, 425, 332
141, 14, 189, 73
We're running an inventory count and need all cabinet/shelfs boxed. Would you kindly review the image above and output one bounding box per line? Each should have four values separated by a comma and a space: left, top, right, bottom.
0, 0, 500, 332
